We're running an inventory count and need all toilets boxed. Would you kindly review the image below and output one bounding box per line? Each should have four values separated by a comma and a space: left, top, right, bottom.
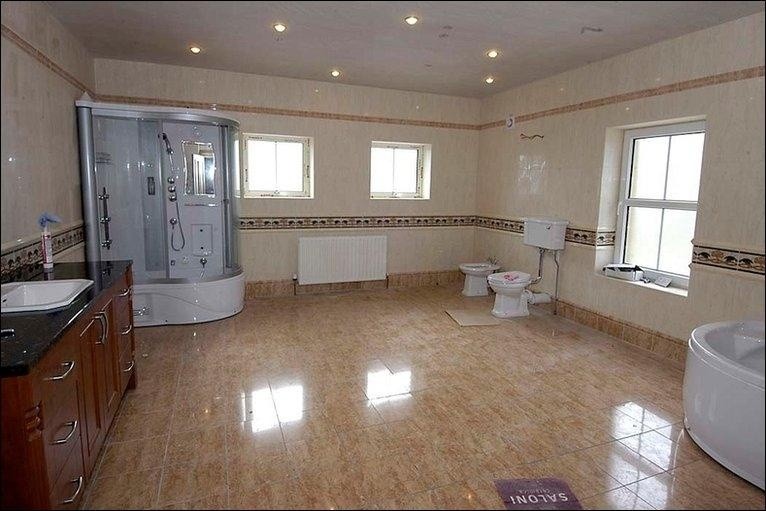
489, 216, 569, 319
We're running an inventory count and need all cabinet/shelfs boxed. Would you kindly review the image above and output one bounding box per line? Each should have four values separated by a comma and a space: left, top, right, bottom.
76, 289, 115, 482
0, 320, 87, 511
116, 266, 138, 407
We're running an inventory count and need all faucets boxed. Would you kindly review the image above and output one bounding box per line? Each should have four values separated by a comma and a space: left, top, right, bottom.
487, 257, 498, 265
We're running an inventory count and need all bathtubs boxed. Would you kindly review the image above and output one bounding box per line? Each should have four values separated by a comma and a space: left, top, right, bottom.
681, 321, 765, 486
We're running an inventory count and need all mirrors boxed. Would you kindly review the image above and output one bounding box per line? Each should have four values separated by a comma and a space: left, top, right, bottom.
180, 139, 216, 198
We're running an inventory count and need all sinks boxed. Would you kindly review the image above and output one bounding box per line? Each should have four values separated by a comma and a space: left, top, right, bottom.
1, 280, 93, 310
459, 262, 500, 278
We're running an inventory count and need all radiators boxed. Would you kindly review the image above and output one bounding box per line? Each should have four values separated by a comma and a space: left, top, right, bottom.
298, 235, 388, 285
